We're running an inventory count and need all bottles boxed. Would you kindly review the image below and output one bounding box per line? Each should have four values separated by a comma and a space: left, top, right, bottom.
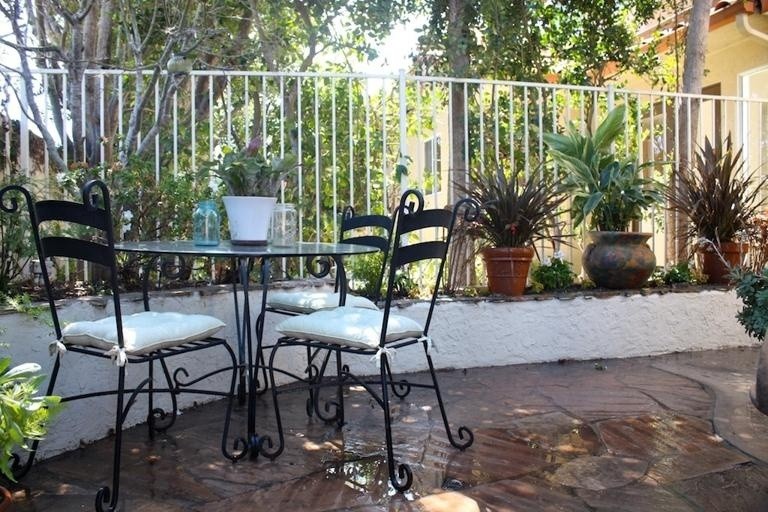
272, 202, 298, 246
192, 198, 221, 245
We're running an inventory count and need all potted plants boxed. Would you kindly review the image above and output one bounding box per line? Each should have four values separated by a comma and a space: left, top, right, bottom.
197, 136, 298, 246
445, 104, 768, 298
721, 265, 768, 417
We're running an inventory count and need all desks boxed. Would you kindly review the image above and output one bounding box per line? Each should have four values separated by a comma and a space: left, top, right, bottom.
113, 239, 380, 459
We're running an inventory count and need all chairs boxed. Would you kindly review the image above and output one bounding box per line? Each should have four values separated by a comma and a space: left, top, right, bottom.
3, 179, 249, 510
258, 191, 476, 492
251, 204, 410, 425
